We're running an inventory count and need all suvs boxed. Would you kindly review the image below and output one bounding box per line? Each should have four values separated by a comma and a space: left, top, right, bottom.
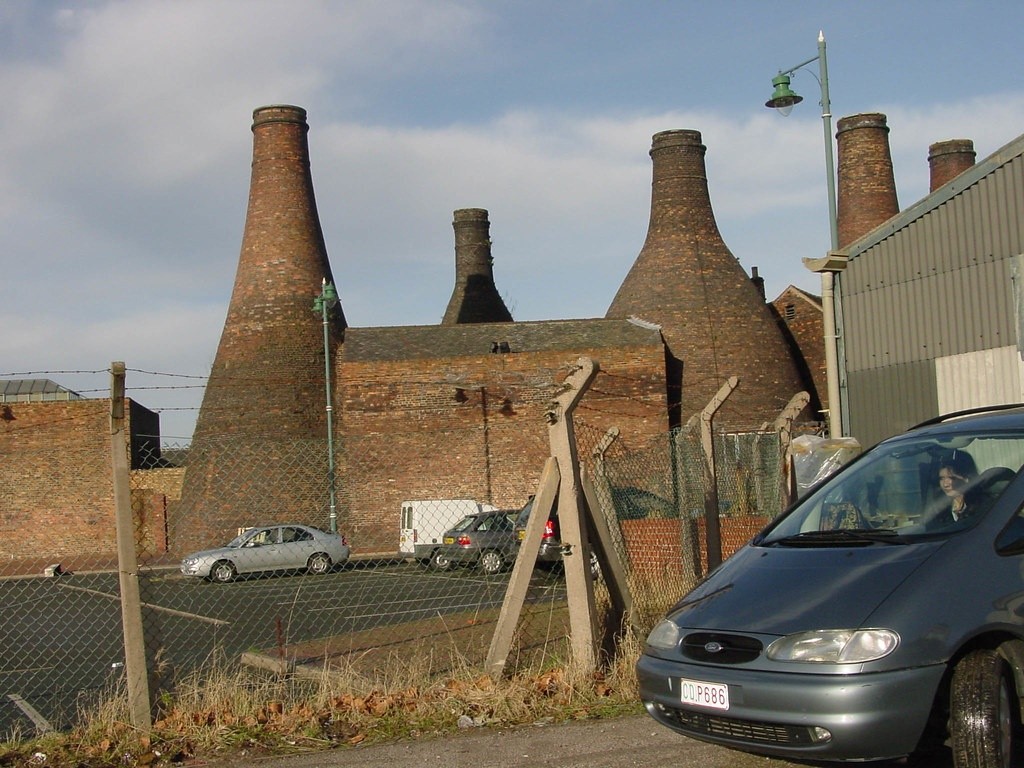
634, 404, 1024, 768
515, 484, 678, 581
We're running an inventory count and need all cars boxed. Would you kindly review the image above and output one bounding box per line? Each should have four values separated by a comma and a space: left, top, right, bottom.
180, 525, 350, 582
687, 498, 760, 521
442, 510, 523, 573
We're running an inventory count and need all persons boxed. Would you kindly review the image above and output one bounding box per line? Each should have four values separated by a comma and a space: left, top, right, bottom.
925, 450, 983, 530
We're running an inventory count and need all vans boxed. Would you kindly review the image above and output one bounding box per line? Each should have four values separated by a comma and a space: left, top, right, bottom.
399, 500, 515, 572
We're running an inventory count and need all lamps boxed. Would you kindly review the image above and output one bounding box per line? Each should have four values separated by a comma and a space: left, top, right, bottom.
450, 386, 481, 404
489, 340, 498, 355
499, 341, 511, 354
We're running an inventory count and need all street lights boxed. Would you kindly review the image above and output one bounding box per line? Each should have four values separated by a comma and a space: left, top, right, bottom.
312, 278, 339, 533
765, 31, 839, 251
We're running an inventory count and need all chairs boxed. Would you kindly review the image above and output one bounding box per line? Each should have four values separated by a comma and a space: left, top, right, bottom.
489, 518, 511, 531
293, 532, 301, 542
264, 530, 273, 544
